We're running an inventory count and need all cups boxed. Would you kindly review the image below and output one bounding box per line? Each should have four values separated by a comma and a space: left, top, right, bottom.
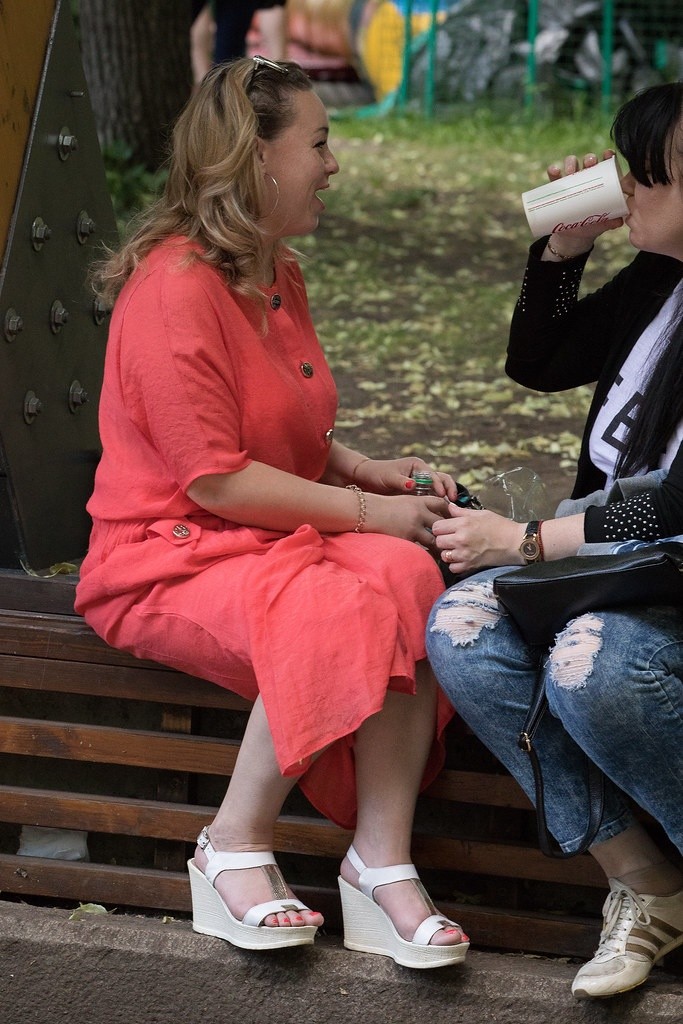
521, 154, 631, 233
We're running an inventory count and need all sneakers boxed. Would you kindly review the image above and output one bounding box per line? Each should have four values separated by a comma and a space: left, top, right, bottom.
571, 878, 683, 1000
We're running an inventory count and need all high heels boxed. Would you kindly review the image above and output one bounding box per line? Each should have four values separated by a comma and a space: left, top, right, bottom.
337, 844, 470, 969
187, 825, 318, 950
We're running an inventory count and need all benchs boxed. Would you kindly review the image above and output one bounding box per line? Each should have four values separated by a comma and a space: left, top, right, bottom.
0, 565, 683, 971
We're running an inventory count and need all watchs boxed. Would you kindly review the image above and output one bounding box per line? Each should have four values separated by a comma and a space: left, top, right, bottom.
519, 520, 540, 564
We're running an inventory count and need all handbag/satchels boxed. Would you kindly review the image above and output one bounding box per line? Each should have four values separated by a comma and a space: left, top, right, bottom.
493, 542, 683, 859
415, 482, 500, 589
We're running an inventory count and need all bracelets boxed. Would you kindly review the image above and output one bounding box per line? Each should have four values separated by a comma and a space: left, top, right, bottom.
547, 235, 573, 259
538, 519, 545, 562
352, 458, 371, 480
345, 484, 367, 533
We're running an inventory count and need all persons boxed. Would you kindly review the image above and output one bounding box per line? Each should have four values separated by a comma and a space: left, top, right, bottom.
72, 57, 471, 967
424, 80, 683, 998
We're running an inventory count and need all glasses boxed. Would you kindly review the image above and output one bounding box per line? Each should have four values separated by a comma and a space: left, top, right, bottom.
247, 55, 288, 95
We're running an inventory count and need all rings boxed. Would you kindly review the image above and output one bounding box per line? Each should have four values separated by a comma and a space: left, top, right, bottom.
445, 551, 454, 562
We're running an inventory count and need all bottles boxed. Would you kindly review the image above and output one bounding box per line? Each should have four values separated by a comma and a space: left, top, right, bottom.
414, 471, 448, 568
